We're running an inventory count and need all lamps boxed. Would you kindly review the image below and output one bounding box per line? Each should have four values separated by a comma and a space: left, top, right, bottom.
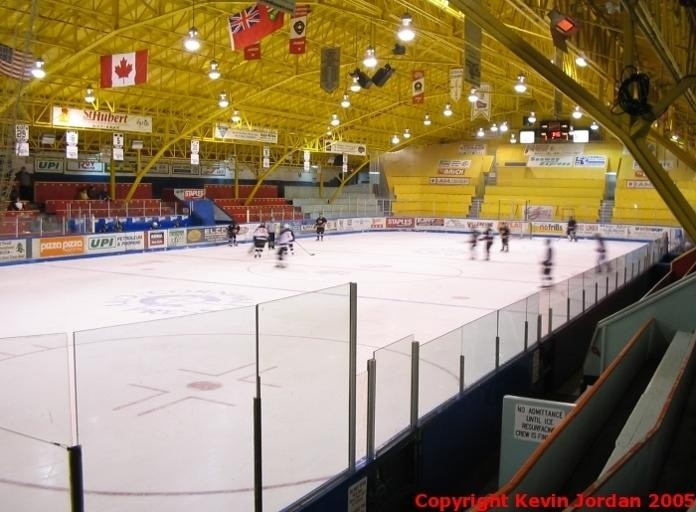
29, 0, 599, 144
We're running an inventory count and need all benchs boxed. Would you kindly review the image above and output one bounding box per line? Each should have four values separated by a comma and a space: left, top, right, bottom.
1, 175, 695, 238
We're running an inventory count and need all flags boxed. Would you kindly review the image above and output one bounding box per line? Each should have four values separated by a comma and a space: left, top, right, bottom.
99, 48, 147, 90
0, 43, 34, 80
229, 2, 284, 51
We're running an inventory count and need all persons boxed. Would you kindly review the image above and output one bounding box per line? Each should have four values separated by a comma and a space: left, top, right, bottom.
315, 212, 327, 241
226, 221, 240, 246
480, 222, 495, 260
466, 226, 480, 260
7, 166, 181, 235
500, 222, 509, 252
252, 216, 296, 267
594, 231, 611, 272
541, 238, 554, 288
566, 216, 577, 242
